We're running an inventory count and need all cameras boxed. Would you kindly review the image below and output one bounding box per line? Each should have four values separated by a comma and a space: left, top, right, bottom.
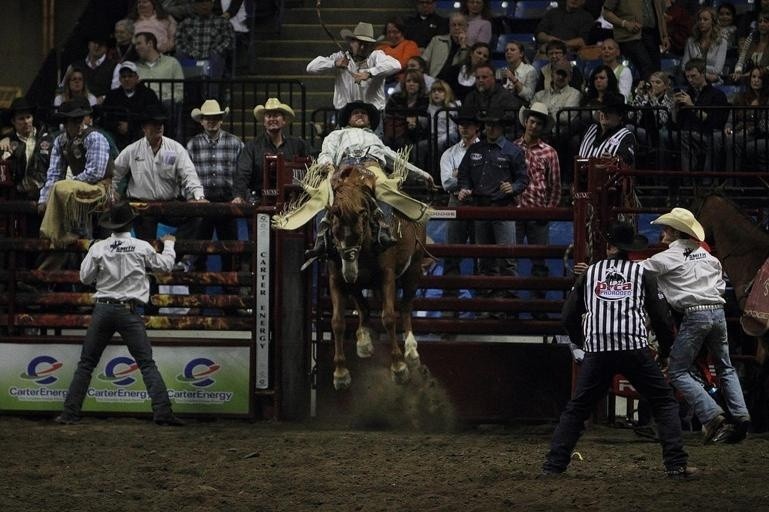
639, 81, 652, 92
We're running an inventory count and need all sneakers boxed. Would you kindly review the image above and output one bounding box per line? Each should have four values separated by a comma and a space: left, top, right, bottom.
155, 413, 187, 426
668, 415, 751, 484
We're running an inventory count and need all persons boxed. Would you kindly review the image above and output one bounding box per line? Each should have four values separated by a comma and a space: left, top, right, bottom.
572, 204, 756, 446
55, 199, 190, 427
2, 2, 767, 328
543, 217, 703, 478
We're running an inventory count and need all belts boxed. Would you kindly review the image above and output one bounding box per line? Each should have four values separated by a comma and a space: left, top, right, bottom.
97, 297, 130, 306
685, 303, 724, 314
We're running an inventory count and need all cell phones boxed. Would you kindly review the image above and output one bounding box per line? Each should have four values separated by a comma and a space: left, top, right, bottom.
674, 87, 680, 94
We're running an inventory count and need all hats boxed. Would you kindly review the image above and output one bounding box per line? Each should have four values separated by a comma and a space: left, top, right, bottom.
341, 22, 386, 44
589, 93, 633, 111
6, 60, 170, 124
191, 99, 230, 122
252, 99, 295, 127
98, 201, 139, 229
448, 57, 572, 134
597, 220, 649, 252
650, 206, 706, 242
341, 101, 381, 132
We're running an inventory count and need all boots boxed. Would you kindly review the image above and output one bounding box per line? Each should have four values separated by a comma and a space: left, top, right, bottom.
304, 207, 331, 258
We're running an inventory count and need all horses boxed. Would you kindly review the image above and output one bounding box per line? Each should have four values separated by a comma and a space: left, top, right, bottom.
682, 177, 769, 433
322, 166, 426, 392
